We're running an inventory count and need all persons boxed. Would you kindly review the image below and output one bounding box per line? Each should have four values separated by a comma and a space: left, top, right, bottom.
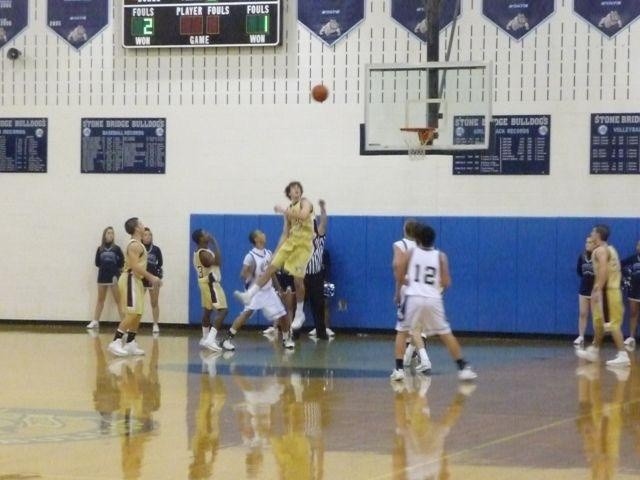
390, 226, 477, 381
140, 229, 163, 334
572, 225, 640, 366
108, 218, 162, 357
86, 227, 125, 330
218, 183, 335, 351
393, 220, 443, 375
190, 229, 228, 351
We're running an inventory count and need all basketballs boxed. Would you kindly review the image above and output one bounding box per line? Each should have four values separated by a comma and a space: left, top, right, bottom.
312, 86, 328, 102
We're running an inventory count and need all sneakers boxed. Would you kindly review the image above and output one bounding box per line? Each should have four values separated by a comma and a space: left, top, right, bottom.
107, 338, 127, 358
390, 382, 477, 398
291, 312, 306, 329
576, 350, 598, 362
606, 356, 631, 366
152, 325, 160, 333
390, 356, 478, 381
624, 337, 635, 345
198, 326, 336, 353
108, 359, 130, 376
577, 363, 628, 379
234, 291, 253, 305
86, 320, 99, 328
123, 339, 145, 356
574, 337, 584, 344
